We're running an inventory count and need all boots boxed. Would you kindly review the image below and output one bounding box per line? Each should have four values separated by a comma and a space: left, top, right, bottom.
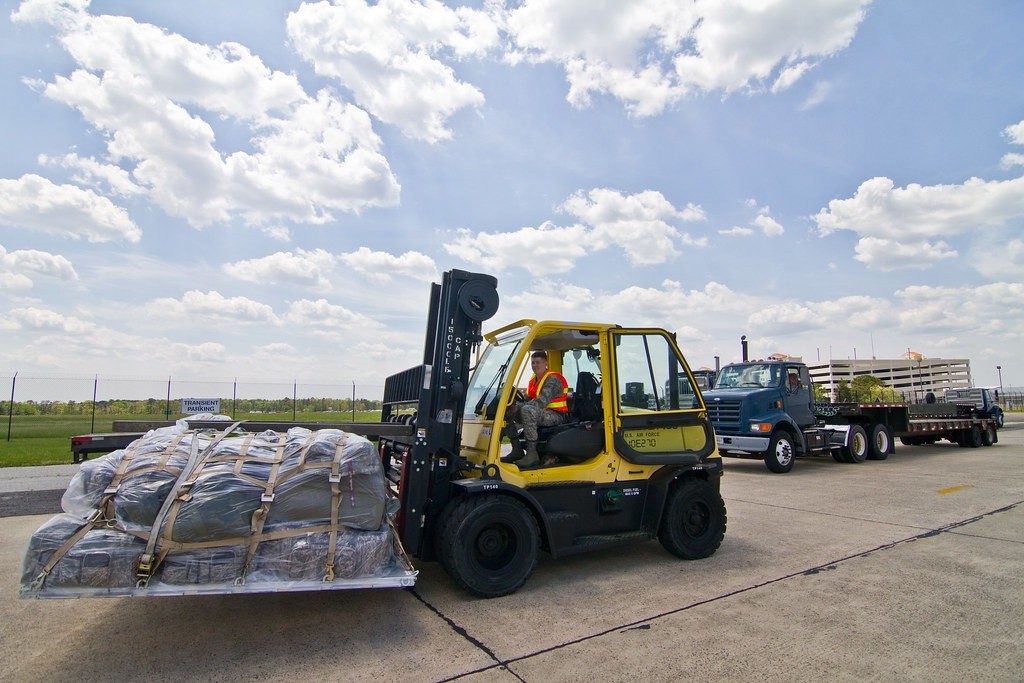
516, 441, 539, 468
500, 440, 524, 463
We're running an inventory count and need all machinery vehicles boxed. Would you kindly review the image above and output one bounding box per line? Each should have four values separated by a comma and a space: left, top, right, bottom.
19, 269, 729, 601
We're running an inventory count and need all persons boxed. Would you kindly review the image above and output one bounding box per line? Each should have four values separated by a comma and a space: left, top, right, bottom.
500, 351, 569, 468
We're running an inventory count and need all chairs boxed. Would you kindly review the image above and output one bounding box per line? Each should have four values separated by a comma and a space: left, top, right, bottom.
790, 373, 798, 385
535, 372, 599, 446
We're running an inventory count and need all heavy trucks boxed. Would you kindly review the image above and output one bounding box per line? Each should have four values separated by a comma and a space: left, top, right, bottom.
659, 335, 1005, 476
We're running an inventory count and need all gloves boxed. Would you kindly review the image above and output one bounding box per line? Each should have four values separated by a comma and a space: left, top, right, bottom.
506, 405, 520, 417
